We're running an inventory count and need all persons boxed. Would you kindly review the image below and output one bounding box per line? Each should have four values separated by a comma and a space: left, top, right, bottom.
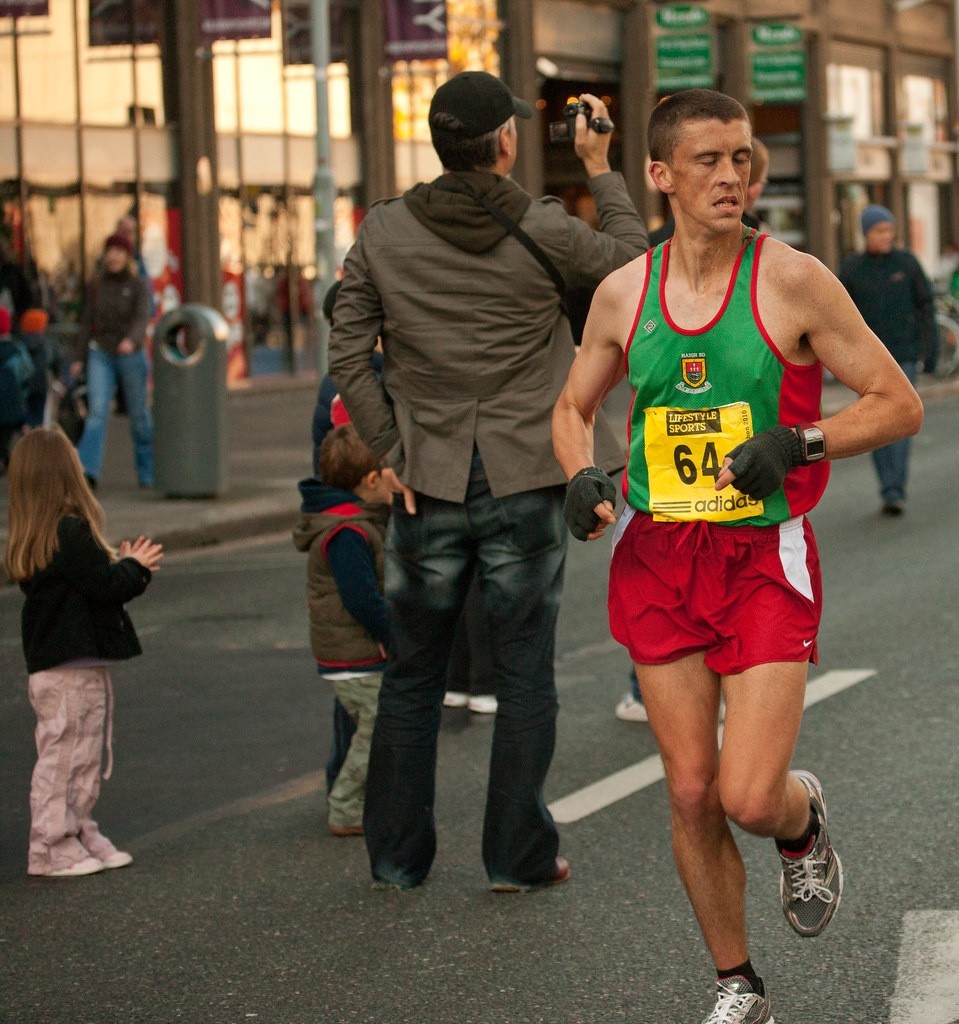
245, 259, 312, 345
293, 279, 497, 836
0, 217, 160, 494
613, 137, 769, 723
326, 70, 651, 891
833, 203, 940, 516
553, 89, 925, 1024
0, 427, 163, 876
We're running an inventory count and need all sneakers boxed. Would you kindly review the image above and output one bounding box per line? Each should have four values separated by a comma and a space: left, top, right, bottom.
775, 770, 844, 938
103, 852, 134, 869
46, 857, 103, 876
700, 976, 774, 1024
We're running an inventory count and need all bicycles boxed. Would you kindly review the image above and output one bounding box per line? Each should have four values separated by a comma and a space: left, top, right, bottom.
933, 297, 959, 376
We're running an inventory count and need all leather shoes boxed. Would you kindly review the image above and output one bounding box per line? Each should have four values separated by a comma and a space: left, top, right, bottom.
548, 856, 572, 885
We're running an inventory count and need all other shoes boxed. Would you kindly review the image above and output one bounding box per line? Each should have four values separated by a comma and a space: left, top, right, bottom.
615, 694, 648, 721
882, 498, 904, 514
468, 694, 498, 714
329, 824, 364, 836
444, 691, 469, 708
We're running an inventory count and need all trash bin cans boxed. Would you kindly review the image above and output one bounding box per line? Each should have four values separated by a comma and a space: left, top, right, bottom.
150, 305, 231, 497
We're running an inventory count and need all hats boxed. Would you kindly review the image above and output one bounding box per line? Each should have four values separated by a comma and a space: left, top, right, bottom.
429, 70, 532, 139
861, 205, 895, 236
105, 236, 134, 258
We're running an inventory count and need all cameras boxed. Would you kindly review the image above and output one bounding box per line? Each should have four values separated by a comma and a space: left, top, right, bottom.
548, 102, 592, 143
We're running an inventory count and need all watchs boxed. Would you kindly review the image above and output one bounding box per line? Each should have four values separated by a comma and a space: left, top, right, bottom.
795, 423, 827, 464
